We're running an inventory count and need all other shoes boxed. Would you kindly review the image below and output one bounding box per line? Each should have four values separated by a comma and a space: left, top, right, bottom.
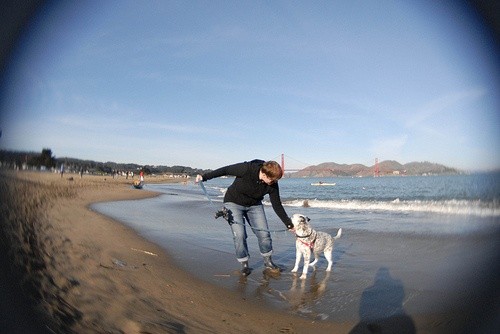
264, 256, 282, 272
241, 262, 249, 276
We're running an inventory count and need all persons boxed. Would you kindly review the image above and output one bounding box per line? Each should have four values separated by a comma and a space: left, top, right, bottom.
60, 161, 145, 185
195, 159, 294, 276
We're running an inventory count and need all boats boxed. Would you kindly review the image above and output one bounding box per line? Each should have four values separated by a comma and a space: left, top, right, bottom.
311, 181, 336, 186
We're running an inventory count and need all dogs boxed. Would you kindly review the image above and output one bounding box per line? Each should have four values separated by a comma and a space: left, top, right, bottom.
290, 214, 342, 279
